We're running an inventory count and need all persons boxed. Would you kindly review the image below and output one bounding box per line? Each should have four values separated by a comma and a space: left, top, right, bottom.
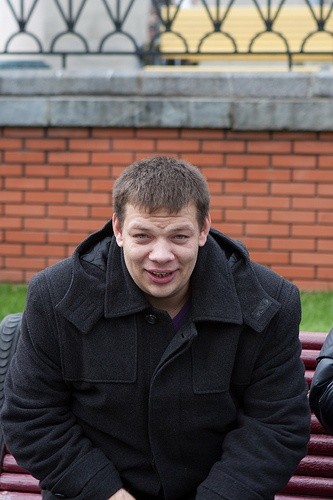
308, 327, 332, 437
0, 156, 313, 500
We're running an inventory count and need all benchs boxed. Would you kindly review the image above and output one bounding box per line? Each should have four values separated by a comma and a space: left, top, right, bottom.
0, 313, 333, 500
